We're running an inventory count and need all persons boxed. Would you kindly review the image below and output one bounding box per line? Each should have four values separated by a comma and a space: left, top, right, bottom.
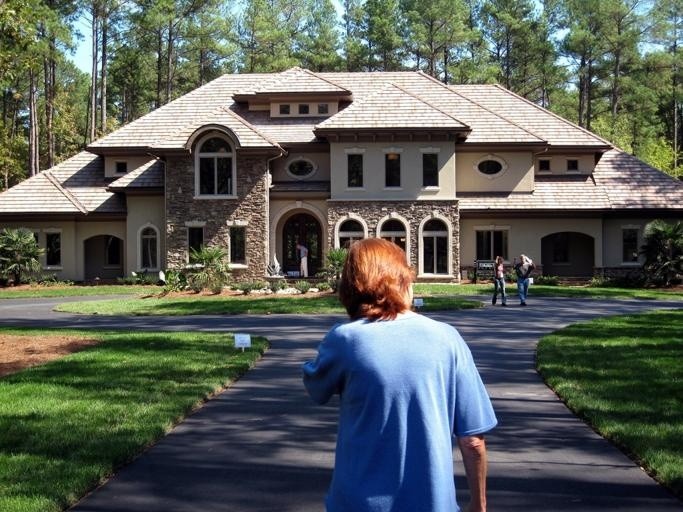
511, 253, 535, 306
491, 254, 506, 307
298, 236, 499, 511
294, 243, 308, 279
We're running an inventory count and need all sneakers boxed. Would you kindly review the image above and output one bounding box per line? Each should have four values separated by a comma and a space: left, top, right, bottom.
502, 303, 507, 306
520, 303, 527, 306
